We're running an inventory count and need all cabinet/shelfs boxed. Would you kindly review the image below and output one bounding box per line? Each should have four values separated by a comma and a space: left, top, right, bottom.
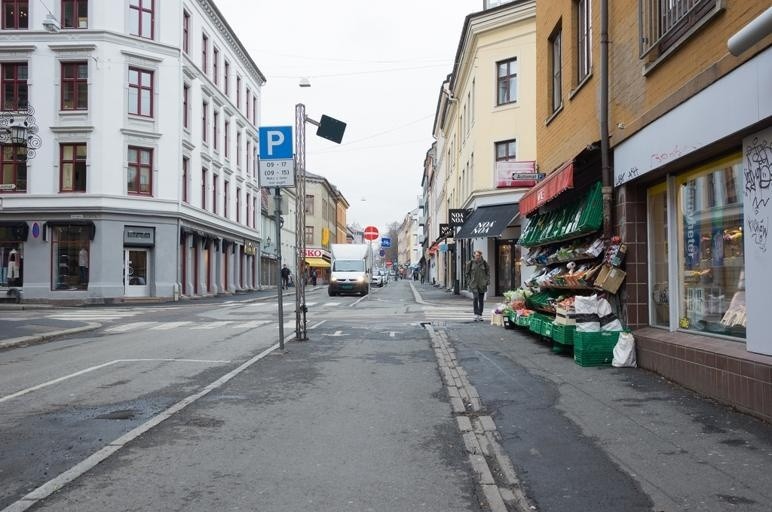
525, 229, 607, 315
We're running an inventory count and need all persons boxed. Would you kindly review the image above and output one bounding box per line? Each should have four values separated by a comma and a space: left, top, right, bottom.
413, 270, 418, 281
312, 270, 317, 286
420, 269, 425, 284
281, 264, 291, 290
304, 269, 307, 285
399, 266, 406, 275
465, 250, 490, 321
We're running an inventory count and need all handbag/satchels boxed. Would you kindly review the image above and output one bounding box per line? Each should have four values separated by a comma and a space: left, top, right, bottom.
611, 332, 638, 368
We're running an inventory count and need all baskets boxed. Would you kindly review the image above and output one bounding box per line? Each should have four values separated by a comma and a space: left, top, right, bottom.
513, 181, 603, 247
489, 288, 623, 370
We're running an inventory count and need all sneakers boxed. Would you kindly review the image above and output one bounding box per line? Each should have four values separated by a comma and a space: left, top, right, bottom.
474, 314, 484, 321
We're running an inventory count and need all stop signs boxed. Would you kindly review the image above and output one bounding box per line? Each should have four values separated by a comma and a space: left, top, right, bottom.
363, 226, 379, 240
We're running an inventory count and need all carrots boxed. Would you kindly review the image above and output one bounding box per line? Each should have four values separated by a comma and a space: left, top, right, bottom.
523, 310, 534, 317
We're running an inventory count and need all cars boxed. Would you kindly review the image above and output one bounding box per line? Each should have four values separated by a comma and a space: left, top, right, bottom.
372, 268, 395, 287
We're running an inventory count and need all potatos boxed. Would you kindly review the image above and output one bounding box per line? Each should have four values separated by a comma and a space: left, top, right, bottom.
560, 295, 575, 306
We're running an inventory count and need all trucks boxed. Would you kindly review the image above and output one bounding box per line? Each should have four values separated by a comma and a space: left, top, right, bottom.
328, 243, 372, 296
